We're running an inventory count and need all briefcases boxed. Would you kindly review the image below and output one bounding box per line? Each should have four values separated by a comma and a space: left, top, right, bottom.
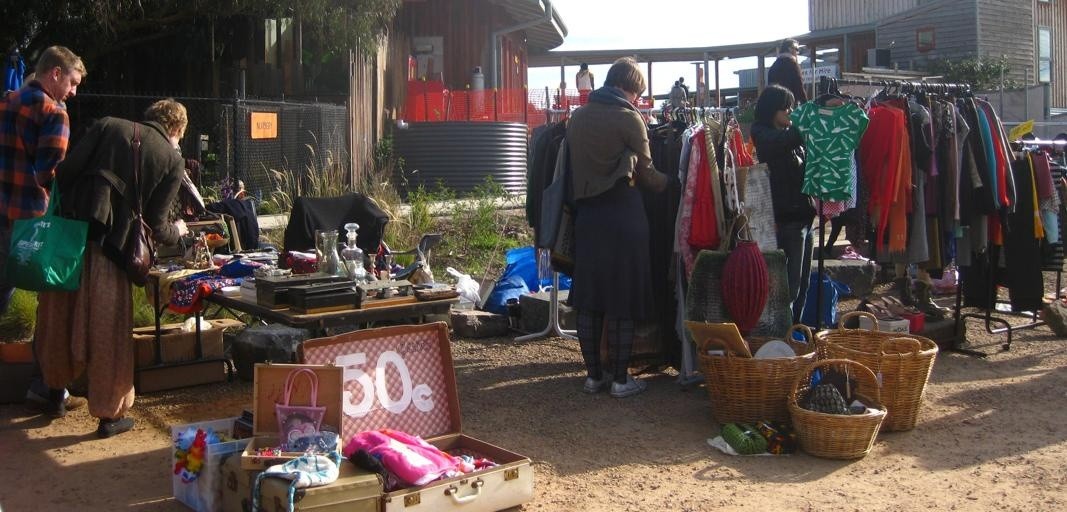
224, 321, 537, 512
299, 320, 535, 512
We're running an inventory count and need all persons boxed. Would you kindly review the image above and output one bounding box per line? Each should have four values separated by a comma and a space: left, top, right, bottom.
41, 96, 190, 439
678, 78, 688, 99
671, 82, 688, 109
0, 46, 91, 412
567, 61, 668, 397
576, 63, 596, 106
766, 54, 818, 160
751, 84, 823, 329
779, 39, 800, 60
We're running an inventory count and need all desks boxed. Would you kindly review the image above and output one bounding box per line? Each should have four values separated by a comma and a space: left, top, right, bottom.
128, 257, 466, 400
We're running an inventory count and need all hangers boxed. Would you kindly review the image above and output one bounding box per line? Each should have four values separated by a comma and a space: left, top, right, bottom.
806, 76, 987, 121
1006, 143, 1067, 166
539, 105, 738, 140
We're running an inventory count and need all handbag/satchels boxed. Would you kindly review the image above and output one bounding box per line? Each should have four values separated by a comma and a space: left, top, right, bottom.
276, 368, 326, 446
125, 219, 153, 287
3, 175, 88, 293
540, 176, 574, 270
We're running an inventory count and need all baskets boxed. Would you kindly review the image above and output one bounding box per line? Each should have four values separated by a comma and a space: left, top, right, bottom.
700, 310, 940, 459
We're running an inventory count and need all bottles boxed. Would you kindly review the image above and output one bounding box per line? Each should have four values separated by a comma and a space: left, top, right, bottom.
318, 231, 340, 275
193, 231, 215, 269
340, 222, 366, 276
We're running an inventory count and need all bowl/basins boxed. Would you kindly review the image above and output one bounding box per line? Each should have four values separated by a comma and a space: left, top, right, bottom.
220, 285, 239, 296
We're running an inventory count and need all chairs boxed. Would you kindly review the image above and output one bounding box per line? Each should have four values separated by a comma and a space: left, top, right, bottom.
198, 200, 278, 325
293, 190, 443, 282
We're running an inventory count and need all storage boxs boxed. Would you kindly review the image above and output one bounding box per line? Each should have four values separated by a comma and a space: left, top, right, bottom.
859, 313, 911, 338
237, 272, 360, 316
166, 414, 261, 511
900, 307, 926, 332
130, 316, 247, 396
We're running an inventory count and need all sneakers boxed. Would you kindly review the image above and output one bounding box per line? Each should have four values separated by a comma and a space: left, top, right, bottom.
44, 397, 67, 418
583, 374, 647, 398
97, 414, 134, 439
24, 388, 88, 411
